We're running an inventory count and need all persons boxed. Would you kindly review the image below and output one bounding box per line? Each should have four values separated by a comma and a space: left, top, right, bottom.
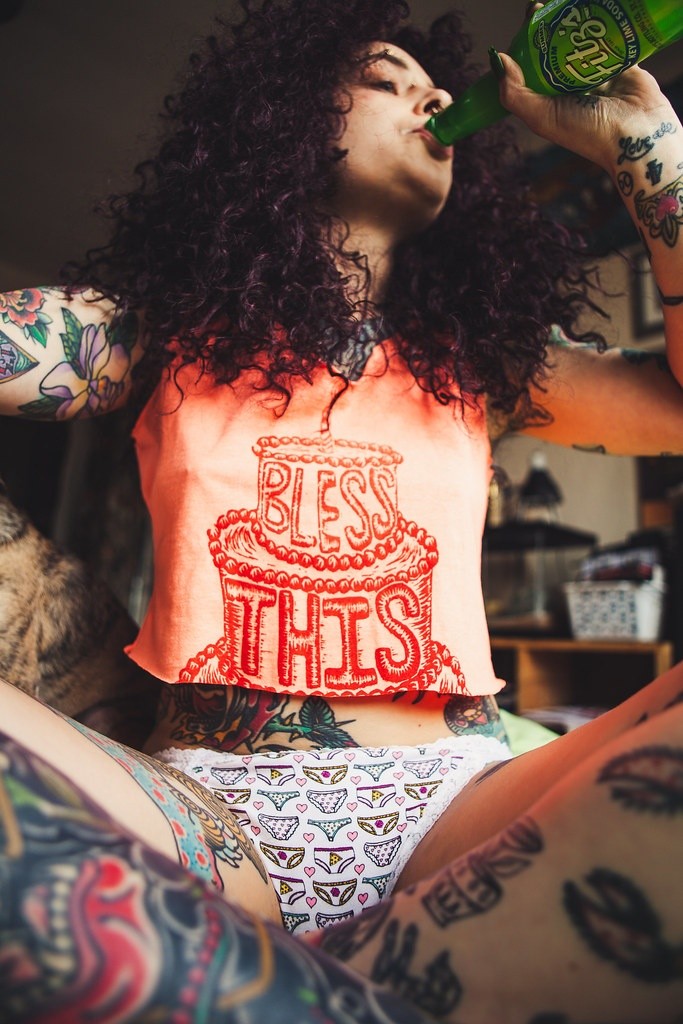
0, 0, 683, 1024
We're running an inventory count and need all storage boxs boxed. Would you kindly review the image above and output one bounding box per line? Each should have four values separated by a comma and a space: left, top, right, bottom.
560, 579, 669, 644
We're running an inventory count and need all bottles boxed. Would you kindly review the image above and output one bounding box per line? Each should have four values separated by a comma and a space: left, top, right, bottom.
482, 450, 565, 529
421, 0, 683, 147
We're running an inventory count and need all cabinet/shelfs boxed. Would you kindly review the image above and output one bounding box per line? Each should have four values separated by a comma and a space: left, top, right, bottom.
487, 638, 676, 739
